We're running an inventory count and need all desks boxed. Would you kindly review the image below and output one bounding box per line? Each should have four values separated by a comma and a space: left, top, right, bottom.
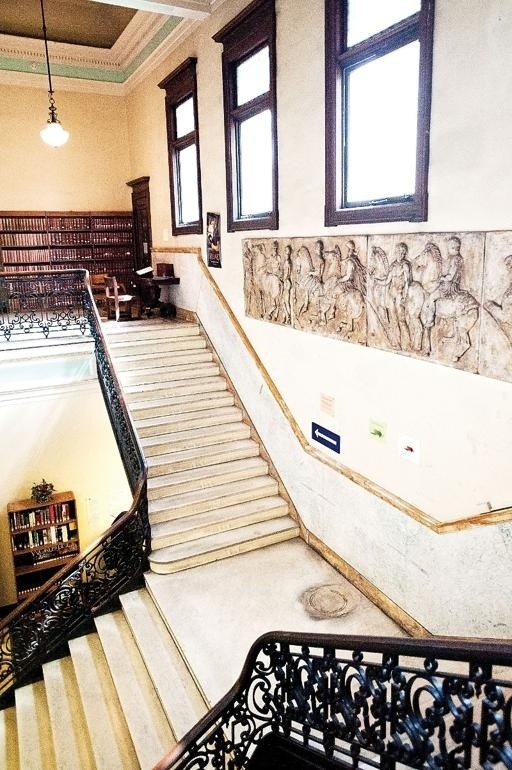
127, 275, 180, 319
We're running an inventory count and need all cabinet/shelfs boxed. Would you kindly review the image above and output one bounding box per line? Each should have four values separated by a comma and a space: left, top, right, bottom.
7, 491, 81, 605
0, 210, 138, 314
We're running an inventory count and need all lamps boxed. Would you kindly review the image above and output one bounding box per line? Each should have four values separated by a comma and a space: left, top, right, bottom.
39, 1, 70, 148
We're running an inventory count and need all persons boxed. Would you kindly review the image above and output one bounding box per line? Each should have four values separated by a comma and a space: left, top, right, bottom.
207, 218, 220, 253
254, 234, 470, 338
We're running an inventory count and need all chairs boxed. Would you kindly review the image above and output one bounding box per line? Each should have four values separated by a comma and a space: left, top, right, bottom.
89, 274, 136, 321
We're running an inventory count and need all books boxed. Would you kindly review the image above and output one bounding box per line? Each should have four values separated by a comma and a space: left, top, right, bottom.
0, 218, 137, 307
9, 501, 78, 601
